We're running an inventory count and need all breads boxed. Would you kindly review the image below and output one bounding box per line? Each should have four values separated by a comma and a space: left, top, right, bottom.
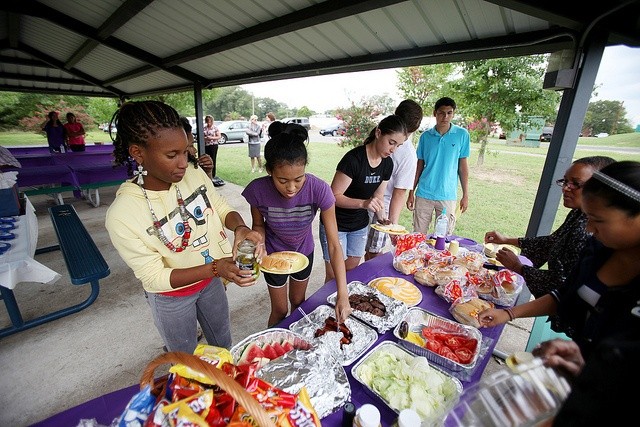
436, 265, 470, 285
414, 264, 441, 287
474, 268, 496, 294
261, 257, 292, 272
393, 251, 423, 276
454, 253, 483, 274
451, 297, 494, 329
492, 270, 524, 307
433, 282, 478, 304
428, 251, 455, 267
272, 252, 297, 260
389, 226, 406, 232
415, 245, 435, 258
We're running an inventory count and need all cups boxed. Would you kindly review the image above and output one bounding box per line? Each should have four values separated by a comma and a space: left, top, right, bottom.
435, 235, 445, 250
448, 240, 459, 256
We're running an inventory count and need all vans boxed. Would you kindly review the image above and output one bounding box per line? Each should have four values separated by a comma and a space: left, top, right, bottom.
280, 117, 311, 133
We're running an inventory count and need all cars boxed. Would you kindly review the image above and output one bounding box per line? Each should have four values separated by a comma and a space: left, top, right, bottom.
319, 125, 341, 137
540, 132, 553, 142
217, 120, 251, 145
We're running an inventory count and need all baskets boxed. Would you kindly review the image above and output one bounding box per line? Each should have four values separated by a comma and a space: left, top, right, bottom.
141, 352, 275, 427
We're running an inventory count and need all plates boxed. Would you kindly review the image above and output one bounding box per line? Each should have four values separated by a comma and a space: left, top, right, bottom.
483, 242, 522, 259
260, 251, 310, 276
369, 224, 409, 236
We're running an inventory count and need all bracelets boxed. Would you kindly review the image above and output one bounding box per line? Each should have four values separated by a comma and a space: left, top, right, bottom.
504, 307, 514, 322
234, 224, 251, 234
211, 260, 220, 277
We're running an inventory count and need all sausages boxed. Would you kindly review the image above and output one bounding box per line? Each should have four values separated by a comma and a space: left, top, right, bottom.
317, 319, 351, 346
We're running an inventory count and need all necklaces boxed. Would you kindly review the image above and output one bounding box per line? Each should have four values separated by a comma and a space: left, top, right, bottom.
143, 181, 190, 252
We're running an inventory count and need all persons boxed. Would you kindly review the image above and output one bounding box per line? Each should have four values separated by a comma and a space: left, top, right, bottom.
63, 113, 85, 151
246, 115, 264, 173
485, 155, 617, 301
265, 113, 274, 143
41, 111, 65, 151
406, 97, 470, 237
365, 99, 423, 261
103, 102, 265, 360
179, 118, 214, 181
203, 115, 221, 177
319, 116, 408, 285
533, 338, 639, 426
241, 121, 351, 328
478, 160, 640, 339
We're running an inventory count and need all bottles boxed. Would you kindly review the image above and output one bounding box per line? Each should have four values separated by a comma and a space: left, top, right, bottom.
434, 207, 448, 236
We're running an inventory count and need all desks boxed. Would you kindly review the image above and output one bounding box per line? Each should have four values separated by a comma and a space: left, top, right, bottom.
29, 234, 535, 427
15, 151, 138, 207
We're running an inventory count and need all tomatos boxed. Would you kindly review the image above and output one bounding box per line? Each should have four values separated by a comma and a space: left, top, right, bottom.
422, 328, 476, 363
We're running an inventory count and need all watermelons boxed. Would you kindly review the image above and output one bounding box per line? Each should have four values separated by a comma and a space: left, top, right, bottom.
238, 341, 264, 366
284, 342, 293, 351
274, 343, 283, 355
262, 343, 277, 359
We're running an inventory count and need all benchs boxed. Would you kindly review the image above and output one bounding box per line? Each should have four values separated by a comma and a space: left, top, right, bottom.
49, 203, 110, 303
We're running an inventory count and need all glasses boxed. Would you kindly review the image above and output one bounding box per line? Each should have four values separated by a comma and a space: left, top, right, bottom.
555, 178, 585, 191
